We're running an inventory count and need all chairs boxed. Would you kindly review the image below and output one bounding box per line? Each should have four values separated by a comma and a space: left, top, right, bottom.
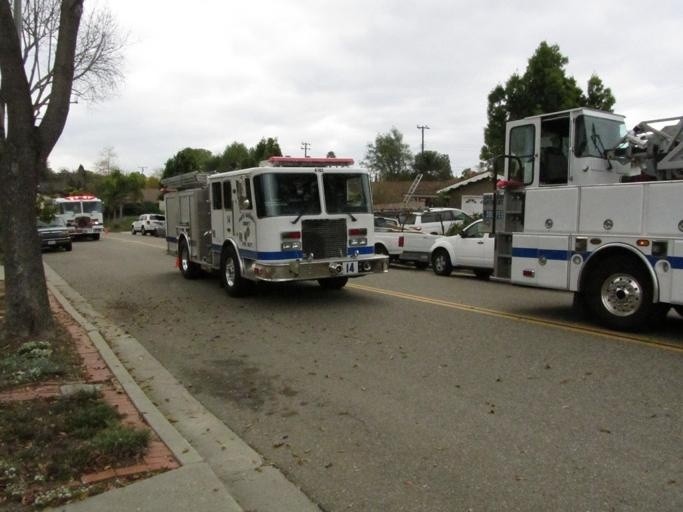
540, 147, 568, 183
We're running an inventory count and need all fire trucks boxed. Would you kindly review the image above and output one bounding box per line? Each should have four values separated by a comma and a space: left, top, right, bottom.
159, 156, 374, 298
492, 107, 681, 333
51, 197, 104, 242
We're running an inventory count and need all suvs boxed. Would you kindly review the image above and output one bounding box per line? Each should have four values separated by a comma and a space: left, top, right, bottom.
131, 214, 165, 236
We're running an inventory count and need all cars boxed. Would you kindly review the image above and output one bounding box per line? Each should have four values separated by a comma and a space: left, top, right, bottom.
396, 207, 477, 236
35, 213, 72, 252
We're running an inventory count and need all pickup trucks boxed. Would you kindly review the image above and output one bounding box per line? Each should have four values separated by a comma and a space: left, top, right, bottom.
374, 217, 445, 271
431, 219, 496, 278
